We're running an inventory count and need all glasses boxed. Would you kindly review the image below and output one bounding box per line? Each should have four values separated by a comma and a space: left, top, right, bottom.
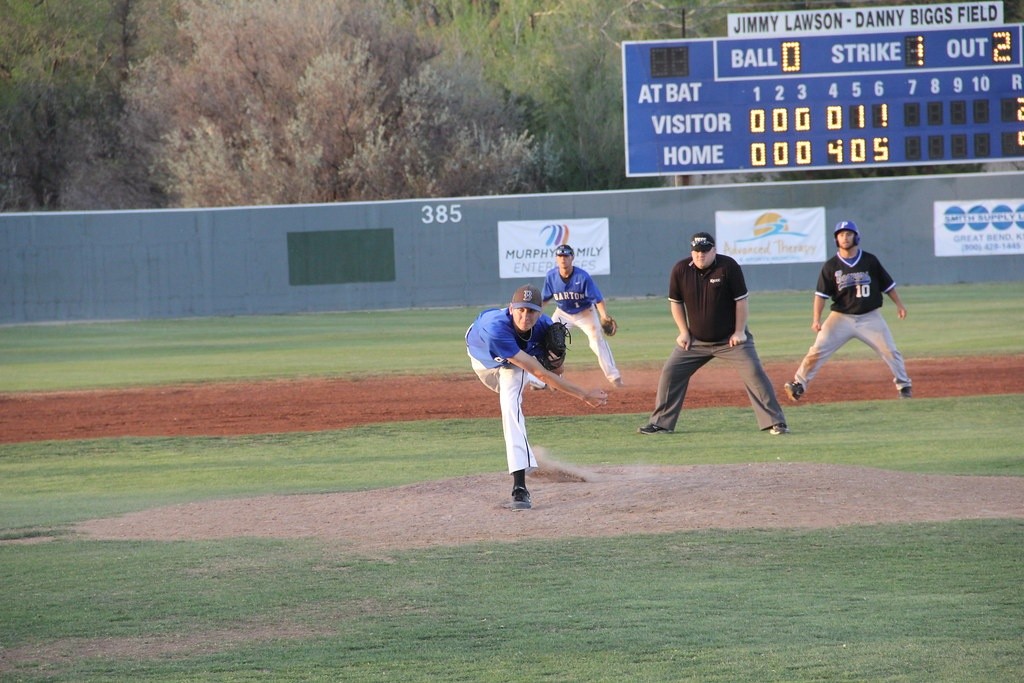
690, 240, 715, 246
556, 248, 572, 255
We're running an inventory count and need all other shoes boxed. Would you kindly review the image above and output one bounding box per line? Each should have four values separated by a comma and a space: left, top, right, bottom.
548, 374, 562, 392
612, 377, 623, 387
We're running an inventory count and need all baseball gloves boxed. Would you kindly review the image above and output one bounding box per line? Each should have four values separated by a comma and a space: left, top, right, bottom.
543, 317, 573, 369
601, 314, 617, 337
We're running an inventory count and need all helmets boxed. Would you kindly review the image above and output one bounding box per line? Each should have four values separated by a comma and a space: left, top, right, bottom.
833, 220, 861, 247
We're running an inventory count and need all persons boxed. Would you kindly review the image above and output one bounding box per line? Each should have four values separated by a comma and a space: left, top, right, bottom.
464, 285, 608, 511
784, 221, 911, 402
540, 244, 624, 388
638, 232, 788, 435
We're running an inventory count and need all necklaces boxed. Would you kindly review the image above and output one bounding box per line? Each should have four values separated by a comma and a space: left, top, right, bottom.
515, 327, 532, 341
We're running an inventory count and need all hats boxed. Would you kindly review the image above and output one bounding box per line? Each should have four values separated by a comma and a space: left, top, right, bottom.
512, 284, 542, 312
689, 232, 715, 252
556, 244, 574, 257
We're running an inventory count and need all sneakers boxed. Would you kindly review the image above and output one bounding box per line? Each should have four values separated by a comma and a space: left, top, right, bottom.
530, 384, 547, 390
899, 387, 912, 399
785, 381, 804, 401
770, 423, 788, 435
511, 485, 532, 511
637, 424, 674, 435
